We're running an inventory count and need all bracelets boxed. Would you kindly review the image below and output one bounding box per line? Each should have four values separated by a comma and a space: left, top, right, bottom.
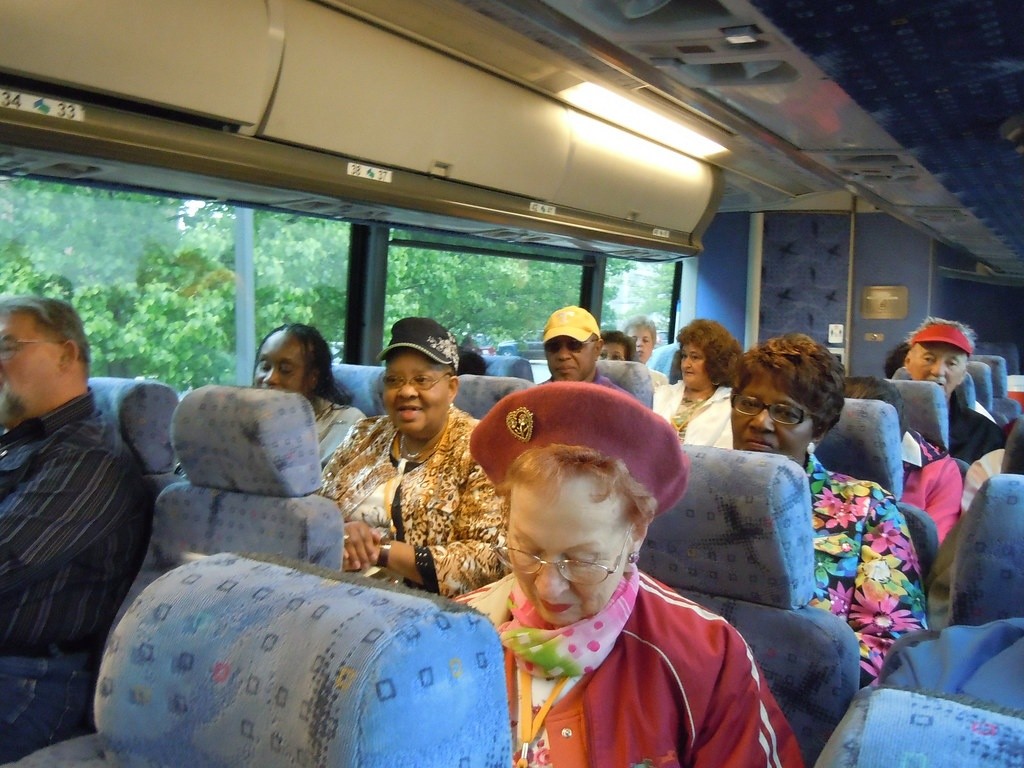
376, 544, 391, 567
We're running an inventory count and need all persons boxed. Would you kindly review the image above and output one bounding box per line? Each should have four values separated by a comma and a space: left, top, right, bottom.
593, 329, 636, 361
884, 315, 1006, 517
169, 324, 367, 477
653, 318, 743, 453
306, 316, 509, 596
448, 381, 804, 768
0, 294, 147, 768
625, 314, 670, 386
537, 305, 636, 397
730, 333, 928, 689
843, 376, 962, 549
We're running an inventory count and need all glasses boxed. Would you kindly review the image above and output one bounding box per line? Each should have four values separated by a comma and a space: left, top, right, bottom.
489, 504, 636, 586
544, 338, 599, 354
383, 372, 454, 391
0, 334, 66, 360
729, 386, 822, 426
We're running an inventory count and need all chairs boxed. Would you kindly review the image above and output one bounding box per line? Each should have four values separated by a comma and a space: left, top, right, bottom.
1, 317, 1024, 768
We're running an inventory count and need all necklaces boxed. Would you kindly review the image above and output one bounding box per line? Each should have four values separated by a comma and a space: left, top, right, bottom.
683, 397, 691, 403
400, 434, 440, 459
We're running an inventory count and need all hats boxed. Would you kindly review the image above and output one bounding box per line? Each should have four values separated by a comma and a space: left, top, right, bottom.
468, 381, 689, 518
542, 305, 601, 345
912, 322, 973, 358
375, 316, 459, 370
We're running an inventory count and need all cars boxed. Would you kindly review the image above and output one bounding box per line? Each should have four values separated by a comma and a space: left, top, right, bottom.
656, 330, 668, 347
495, 329, 548, 361
